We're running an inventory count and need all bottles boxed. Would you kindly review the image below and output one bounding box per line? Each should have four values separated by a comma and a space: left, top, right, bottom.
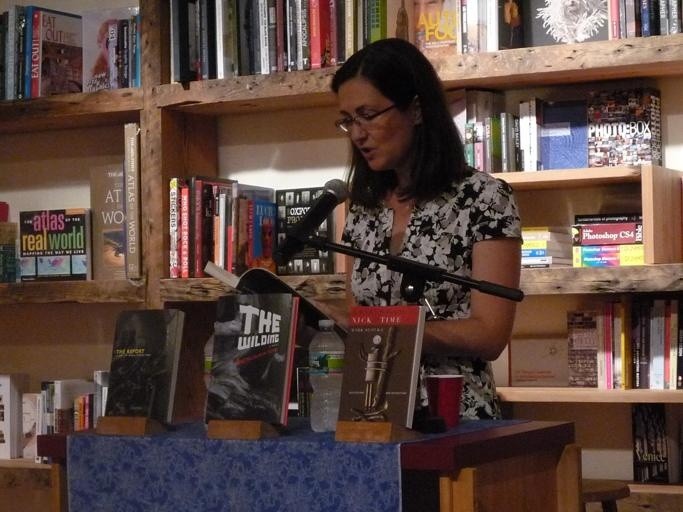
307, 320, 348, 431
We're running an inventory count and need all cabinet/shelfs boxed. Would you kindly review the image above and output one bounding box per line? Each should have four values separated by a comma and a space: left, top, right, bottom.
1, 0, 682, 512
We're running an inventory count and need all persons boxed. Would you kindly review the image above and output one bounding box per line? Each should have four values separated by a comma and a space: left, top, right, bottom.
306, 38, 524, 421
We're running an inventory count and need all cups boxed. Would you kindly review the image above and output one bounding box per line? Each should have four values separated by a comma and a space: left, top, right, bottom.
423, 376, 465, 429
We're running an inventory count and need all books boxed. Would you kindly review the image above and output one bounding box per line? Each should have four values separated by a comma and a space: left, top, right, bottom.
1, 294, 426, 465
566, 300, 682, 485
1, 6, 142, 101
445, 88, 662, 268
170, 175, 349, 336
171, 2, 682, 82
2, 123, 140, 283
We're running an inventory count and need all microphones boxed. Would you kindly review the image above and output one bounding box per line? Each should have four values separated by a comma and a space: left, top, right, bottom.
272, 178, 349, 266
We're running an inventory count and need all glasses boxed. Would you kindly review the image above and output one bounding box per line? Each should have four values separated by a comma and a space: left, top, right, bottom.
335, 105, 397, 135
